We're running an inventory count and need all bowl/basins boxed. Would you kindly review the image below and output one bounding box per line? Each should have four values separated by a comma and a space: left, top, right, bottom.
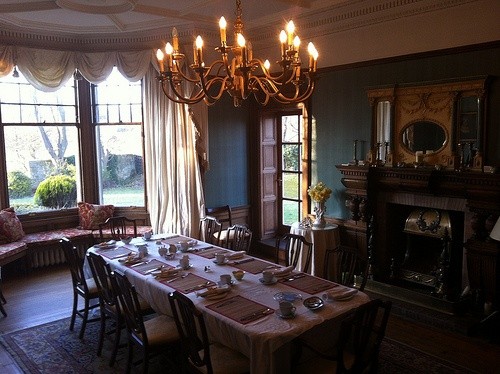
121, 238, 131, 244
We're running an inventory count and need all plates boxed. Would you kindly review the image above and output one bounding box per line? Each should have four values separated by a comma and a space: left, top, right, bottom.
274, 309, 296, 317
259, 277, 277, 284
303, 296, 323, 310
213, 260, 229, 264
273, 291, 302, 302
335, 293, 354, 300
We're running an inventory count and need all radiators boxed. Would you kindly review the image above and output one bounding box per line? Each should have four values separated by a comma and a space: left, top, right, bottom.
33, 238, 101, 267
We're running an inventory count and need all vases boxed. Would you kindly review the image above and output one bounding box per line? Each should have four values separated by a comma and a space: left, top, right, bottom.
312, 199, 326, 227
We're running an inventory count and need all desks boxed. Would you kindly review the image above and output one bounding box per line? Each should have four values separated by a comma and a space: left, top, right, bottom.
288, 222, 341, 285
85, 233, 376, 374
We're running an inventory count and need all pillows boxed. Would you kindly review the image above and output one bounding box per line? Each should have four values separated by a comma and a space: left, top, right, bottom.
0, 206, 25, 243
77, 202, 115, 229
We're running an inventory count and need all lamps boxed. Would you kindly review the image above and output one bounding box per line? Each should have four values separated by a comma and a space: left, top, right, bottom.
157, 0, 319, 107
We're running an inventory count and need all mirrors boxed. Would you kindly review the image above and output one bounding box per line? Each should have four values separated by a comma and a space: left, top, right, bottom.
399, 118, 448, 156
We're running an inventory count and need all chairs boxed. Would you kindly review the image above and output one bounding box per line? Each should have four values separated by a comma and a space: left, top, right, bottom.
59, 205, 392, 374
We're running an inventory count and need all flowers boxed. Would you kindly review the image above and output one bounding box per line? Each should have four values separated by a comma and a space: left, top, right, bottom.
307, 182, 333, 202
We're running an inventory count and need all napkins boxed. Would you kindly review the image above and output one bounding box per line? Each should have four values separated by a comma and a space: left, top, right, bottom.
175, 239, 198, 246
195, 285, 231, 298
223, 250, 246, 258
118, 255, 142, 263
151, 267, 184, 276
321, 289, 358, 302
274, 265, 294, 275
98, 241, 116, 248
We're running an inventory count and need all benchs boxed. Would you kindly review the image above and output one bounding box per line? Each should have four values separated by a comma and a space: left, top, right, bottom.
0, 239, 28, 266
93, 224, 153, 246
20, 225, 92, 249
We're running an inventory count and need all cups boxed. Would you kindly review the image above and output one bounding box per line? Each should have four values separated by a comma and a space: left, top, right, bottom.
278, 302, 296, 315
215, 253, 224, 262
220, 275, 231, 285
263, 271, 272, 282
232, 270, 245, 280
144, 233, 151, 239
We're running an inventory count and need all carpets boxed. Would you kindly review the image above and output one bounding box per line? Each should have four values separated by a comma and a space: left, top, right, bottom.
0, 304, 483, 374
